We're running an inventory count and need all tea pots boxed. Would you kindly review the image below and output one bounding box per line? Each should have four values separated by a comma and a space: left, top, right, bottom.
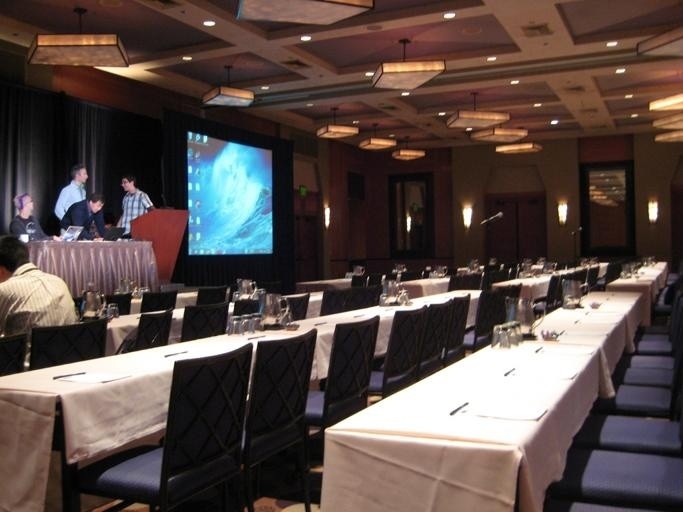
378, 279, 405, 306
542, 261, 559, 273
504, 296, 546, 340
237, 278, 258, 297
562, 279, 582, 310
435, 264, 448, 277
352, 266, 365, 276
257, 292, 290, 330
79, 289, 106, 321
642, 255, 655, 268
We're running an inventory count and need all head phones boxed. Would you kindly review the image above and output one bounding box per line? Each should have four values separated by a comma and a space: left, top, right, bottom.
15, 193, 28, 210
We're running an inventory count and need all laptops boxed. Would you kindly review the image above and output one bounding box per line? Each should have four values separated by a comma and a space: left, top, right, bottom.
103, 228, 126, 241
61, 226, 85, 240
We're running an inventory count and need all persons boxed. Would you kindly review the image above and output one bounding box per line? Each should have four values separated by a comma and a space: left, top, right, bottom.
0, 235, 78, 371
60, 192, 108, 242
54, 164, 90, 222
8, 193, 63, 246
116, 173, 157, 240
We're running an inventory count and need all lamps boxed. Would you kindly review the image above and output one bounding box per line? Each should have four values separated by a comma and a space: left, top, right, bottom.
446, 92, 510, 128
371, 38, 446, 90
358, 124, 397, 150
316, 107, 360, 138
201, 64, 255, 108
391, 135, 426, 160
469, 124, 528, 143
233, 0, 375, 25
638, 25, 683, 143
495, 138, 544, 154
26, 8, 129, 67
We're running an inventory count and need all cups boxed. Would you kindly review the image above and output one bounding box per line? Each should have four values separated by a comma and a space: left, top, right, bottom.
120, 279, 134, 293
491, 319, 522, 351
226, 312, 264, 338
620, 262, 638, 279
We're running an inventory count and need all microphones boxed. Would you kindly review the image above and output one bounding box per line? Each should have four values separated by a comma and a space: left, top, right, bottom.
481, 212, 504, 225
571, 227, 582, 235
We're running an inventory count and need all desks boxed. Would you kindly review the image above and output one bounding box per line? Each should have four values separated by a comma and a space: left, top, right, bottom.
26, 239, 161, 296
